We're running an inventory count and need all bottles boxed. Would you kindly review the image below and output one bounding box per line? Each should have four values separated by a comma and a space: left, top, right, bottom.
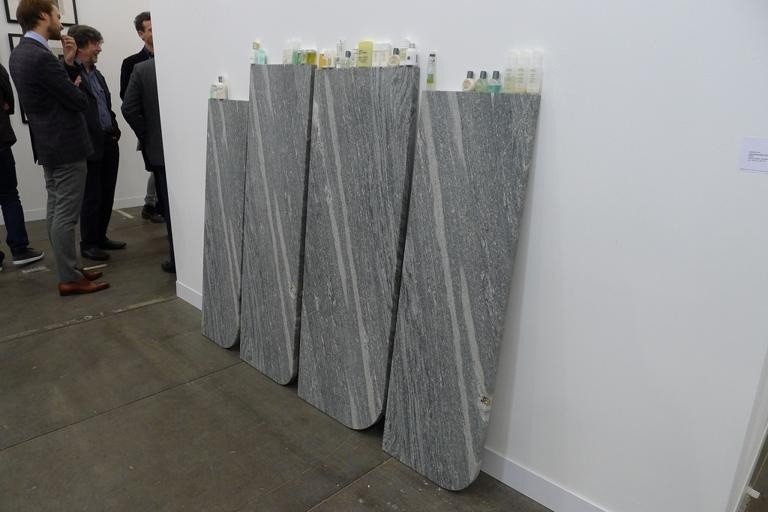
343, 50, 354, 69
425, 54, 438, 90
215, 76, 227, 101
387, 41, 418, 67
462, 66, 539, 93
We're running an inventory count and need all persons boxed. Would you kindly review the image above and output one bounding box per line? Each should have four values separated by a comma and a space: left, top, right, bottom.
120, 11, 166, 224
120, 57, 176, 274
58, 24, 128, 261
8, 0, 110, 297
0, 63, 46, 272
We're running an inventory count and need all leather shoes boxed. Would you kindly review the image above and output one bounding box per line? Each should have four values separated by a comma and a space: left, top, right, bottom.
58, 269, 110, 297
80, 239, 127, 261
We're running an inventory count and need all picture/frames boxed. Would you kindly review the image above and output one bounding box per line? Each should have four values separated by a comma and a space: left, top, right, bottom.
4, 0, 78, 123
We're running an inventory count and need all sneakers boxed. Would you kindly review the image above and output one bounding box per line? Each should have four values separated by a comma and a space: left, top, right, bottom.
12, 247, 45, 265
142, 202, 166, 223
160, 260, 176, 273
